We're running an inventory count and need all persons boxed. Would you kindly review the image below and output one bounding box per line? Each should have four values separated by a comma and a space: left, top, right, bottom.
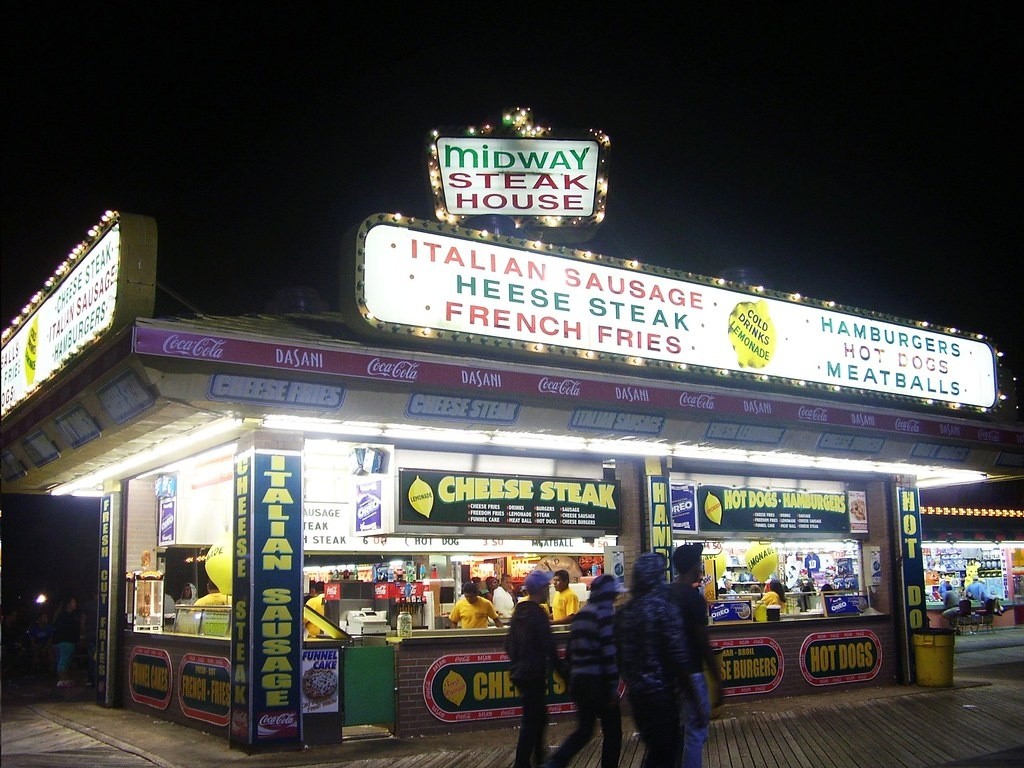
304, 580, 326, 638
718, 577, 833, 614
174, 578, 232, 606
667, 543, 724, 768
548, 575, 630, 768
612, 553, 690, 768
937, 576, 1003, 635
503, 572, 565, 767
449, 574, 550, 627
549, 569, 579, 624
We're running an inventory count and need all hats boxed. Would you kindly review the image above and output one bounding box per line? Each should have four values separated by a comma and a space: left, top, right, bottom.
945, 577, 950, 581
526, 569, 553, 593
973, 576, 978, 580
673, 543, 702, 570
590, 573, 631, 599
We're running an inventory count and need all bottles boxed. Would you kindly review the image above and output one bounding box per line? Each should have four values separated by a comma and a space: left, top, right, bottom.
755, 601, 767, 621
406, 581, 411, 596
431, 564, 437, 579
406, 562, 413, 582
397, 565, 403, 581
333, 565, 359, 581
592, 563, 601, 576
397, 612, 412, 637
420, 564, 426, 580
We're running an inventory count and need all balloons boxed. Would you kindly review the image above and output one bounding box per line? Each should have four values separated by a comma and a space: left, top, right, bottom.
745, 543, 777, 583
702, 549, 725, 582
204, 531, 234, 596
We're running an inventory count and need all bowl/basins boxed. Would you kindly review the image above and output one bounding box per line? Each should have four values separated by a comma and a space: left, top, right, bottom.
788, 607, 801, 614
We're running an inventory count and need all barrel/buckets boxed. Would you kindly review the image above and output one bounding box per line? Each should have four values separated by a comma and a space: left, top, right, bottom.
766, 605, 782, 622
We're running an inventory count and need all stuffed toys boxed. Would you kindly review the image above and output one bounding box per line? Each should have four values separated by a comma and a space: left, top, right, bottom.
964, 563, 984, 588
926, 571, 940, 586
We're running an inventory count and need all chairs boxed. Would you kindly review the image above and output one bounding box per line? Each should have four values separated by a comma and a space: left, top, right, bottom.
975, 598, 996, 634
955, 600, 974, 635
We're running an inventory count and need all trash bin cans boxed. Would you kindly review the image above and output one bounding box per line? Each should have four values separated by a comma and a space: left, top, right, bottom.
912, 627, 956, 687
